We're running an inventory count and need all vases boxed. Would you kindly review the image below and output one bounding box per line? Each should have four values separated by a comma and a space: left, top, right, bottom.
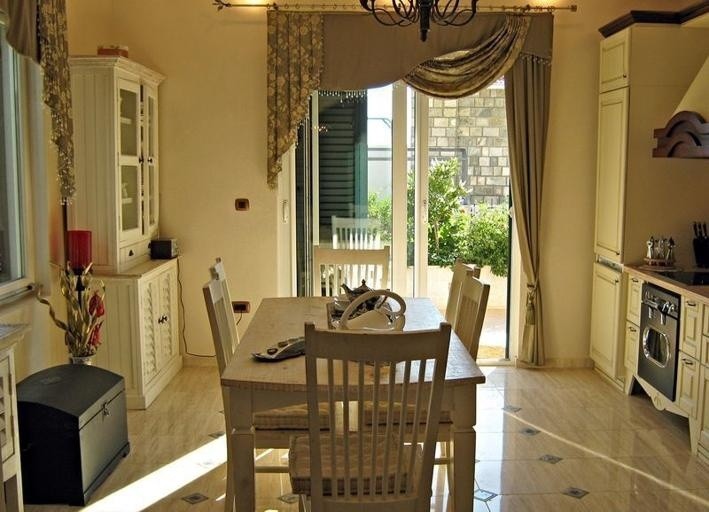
70, 355, 94, 366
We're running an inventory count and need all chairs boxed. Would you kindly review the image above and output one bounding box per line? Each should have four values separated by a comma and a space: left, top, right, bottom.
287, 321, 452, 512
202, 258, 333, 511
328, 217, 380, 299
309, 246, 392, 301
363, 270, 489, 496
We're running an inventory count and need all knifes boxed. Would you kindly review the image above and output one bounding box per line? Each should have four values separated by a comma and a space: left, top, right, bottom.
691, 220, 708, 268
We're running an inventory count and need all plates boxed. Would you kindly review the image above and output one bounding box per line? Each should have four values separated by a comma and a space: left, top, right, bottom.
326, 300, 396, 330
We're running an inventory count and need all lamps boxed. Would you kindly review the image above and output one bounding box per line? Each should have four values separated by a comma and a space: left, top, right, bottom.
63, 230, 94, 311
355, 0, 481, 42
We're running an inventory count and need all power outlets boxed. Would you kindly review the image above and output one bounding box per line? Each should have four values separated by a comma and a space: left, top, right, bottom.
231, 301, 250, 315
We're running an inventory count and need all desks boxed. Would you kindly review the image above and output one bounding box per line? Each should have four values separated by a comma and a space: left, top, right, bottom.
221, 297, 486, 512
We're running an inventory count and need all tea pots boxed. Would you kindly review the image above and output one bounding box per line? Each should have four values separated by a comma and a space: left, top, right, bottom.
339, 279, 381, 312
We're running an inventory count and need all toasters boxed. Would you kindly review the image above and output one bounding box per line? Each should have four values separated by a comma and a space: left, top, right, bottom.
150, 238, 179, 260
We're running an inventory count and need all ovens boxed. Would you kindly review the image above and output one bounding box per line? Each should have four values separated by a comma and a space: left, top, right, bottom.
637, 283, 680, 402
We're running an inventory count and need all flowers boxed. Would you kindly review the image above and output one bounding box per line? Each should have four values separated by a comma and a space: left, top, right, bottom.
27, 258, 106, 357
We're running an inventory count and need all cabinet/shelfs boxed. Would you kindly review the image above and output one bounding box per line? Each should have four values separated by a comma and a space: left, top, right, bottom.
589, 256, 648, 394
599, 9, 709, 94
65, 55, 166, 275
60, 257, 183, 411
625, 264, 698, 459
700, 294, 709, 473
1, 324, 29, 512
594, 87, 709, 259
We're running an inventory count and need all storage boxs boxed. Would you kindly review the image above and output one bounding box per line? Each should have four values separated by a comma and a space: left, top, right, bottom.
17, 362, 131, 506
